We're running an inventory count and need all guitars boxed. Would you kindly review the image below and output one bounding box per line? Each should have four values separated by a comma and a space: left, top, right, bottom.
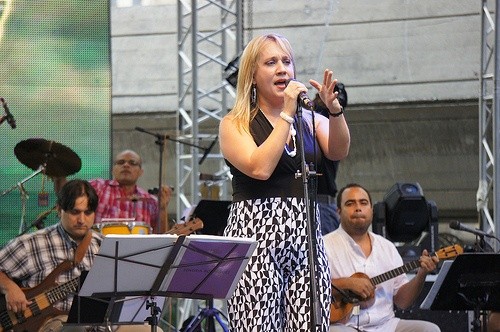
5, 216, 203, 332
330, 244, 465, 324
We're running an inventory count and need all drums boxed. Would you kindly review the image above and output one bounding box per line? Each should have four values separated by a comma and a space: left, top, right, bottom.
91, 220, 154, 237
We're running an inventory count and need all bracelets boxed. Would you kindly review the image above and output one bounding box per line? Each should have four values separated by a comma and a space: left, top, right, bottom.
327, 106, 343, 117
280, 111, 295, 123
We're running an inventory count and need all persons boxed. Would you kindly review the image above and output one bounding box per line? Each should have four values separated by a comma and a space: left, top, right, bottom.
218, 33, 351, 332
321, 184, 442, 332
0, 178, 163, 332
314, 81, 347, 236
53, 148, 171, 234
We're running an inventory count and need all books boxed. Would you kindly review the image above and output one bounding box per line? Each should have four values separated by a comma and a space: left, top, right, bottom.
77, 234, 258, 300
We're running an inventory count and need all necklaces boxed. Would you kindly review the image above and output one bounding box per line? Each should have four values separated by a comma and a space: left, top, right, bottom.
259, 107, 297, 157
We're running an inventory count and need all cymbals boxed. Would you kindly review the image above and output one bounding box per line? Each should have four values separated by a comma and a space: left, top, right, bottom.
14, 137, 83, 178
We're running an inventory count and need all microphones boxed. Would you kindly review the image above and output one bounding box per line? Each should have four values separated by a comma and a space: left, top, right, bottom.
1, 98, 16, 129
450, 220, 492, 238
199, 136, 218, 164
286, 79, 313, 109
